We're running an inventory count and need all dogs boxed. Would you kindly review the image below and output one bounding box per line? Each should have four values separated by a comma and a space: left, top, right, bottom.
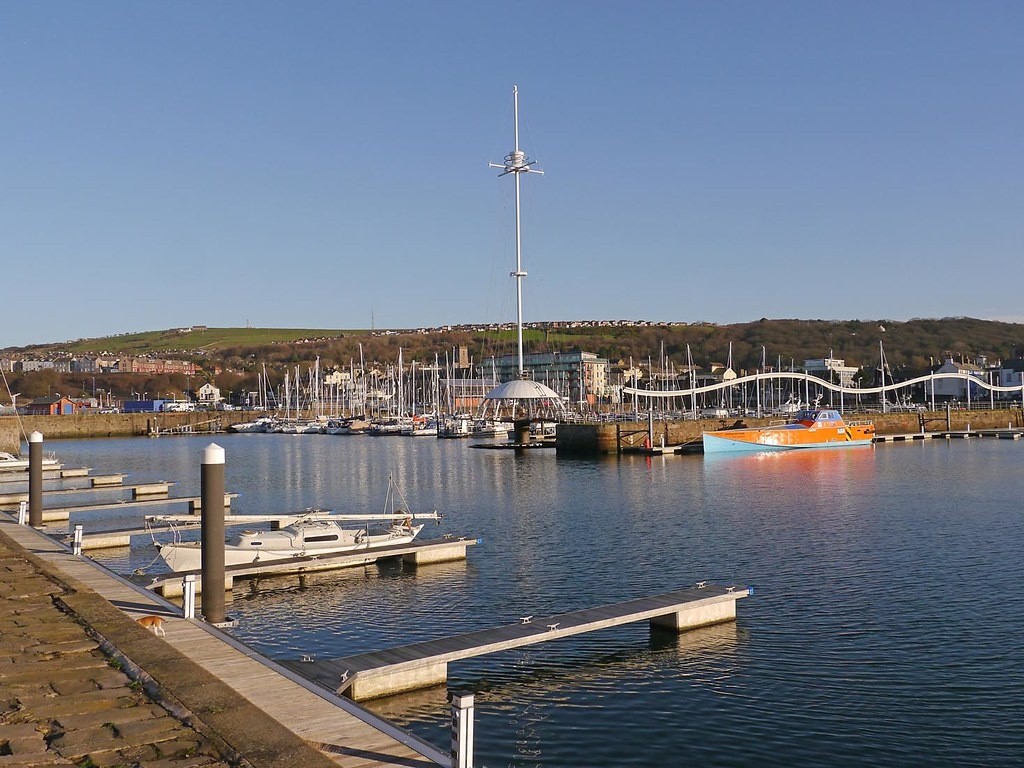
137, 616, 166, 638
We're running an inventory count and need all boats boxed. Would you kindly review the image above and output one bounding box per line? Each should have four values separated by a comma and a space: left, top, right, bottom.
231, 340, 706, 440
703, 395, 875, 452
719, 341, 931, 418
145, 473, 444, 572
